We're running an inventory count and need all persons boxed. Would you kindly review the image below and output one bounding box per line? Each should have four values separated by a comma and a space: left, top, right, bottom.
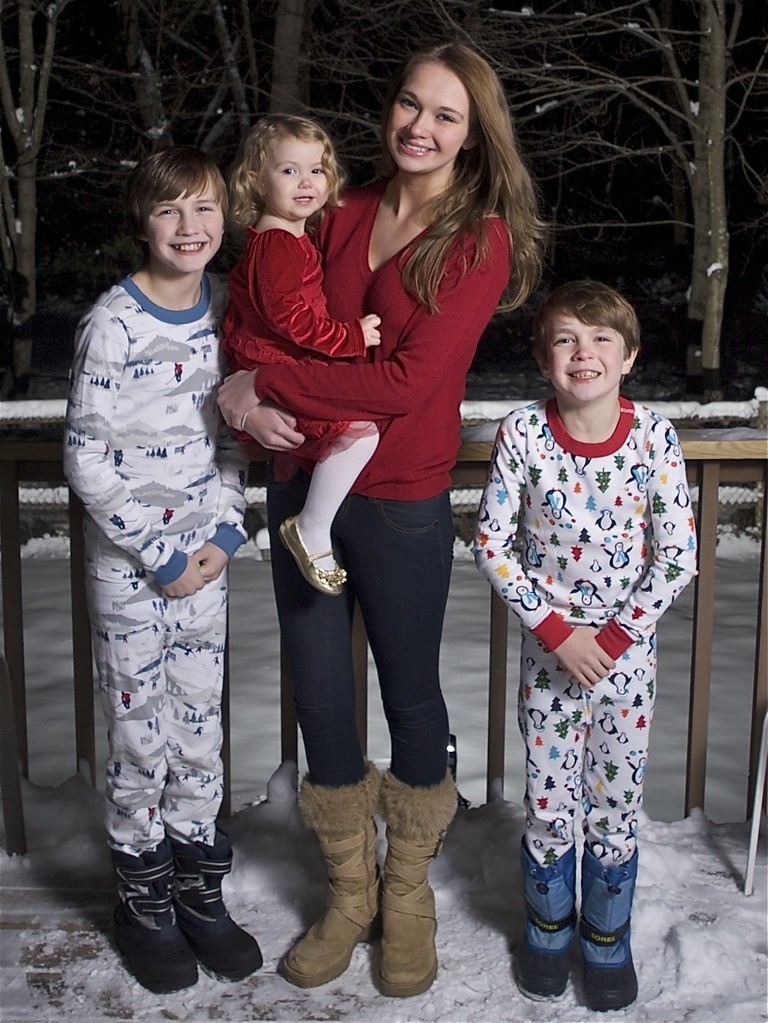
216, 41, 543, 997
470, 279, 699, 1013
65, 147, 264, 993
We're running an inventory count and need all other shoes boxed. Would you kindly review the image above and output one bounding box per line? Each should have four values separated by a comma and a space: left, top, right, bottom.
276, 515, 346, 597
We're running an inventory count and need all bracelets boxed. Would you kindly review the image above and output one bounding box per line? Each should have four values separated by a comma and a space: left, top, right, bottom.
241, 412, 248, 428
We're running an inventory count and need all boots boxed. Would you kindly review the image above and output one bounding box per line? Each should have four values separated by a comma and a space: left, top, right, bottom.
516, 834, 576, 1002
377, 767, 458, 997
579, 841, 639, 1013
164, 826, 263, 983
282, 760, 383, 988
105, 836, 199, 994
